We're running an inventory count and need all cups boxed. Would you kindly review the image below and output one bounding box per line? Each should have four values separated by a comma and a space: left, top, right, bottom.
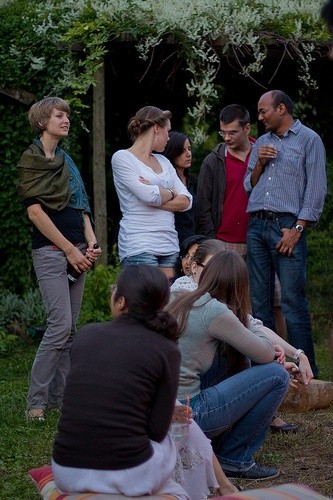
171, 422, 191, 470
264, 142, 277, 158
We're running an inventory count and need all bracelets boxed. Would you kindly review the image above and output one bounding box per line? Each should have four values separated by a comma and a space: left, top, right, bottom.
292, 348, 306, 363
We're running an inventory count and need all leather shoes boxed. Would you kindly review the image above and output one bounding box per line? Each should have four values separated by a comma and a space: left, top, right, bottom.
269, 422, 299, 435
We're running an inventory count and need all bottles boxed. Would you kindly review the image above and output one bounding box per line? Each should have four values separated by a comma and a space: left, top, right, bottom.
68, 242, 100, 287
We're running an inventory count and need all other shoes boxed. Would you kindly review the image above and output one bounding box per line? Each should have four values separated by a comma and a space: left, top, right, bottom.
222, 463, 280, 480
25, 410, 46, 424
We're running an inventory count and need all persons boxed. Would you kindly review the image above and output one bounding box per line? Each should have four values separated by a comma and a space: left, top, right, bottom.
48, 262, 248, 500
163, 231, 315, 483
110, 106, 195, 278
193, 104, 260, 266
242, 89, 329, 381
14, 96, 102, 418
161, 130, 194, 245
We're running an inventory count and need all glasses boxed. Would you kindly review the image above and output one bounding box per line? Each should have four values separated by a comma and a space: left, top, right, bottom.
180, 255, 194, 261
218, 130, 241, 136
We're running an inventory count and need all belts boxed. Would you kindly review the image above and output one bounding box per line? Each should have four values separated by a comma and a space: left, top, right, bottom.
251, 210, 292, 220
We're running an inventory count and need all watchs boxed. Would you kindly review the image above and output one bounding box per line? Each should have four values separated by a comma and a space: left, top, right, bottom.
165, 188, 177, 200
292, 223, 305, 234
